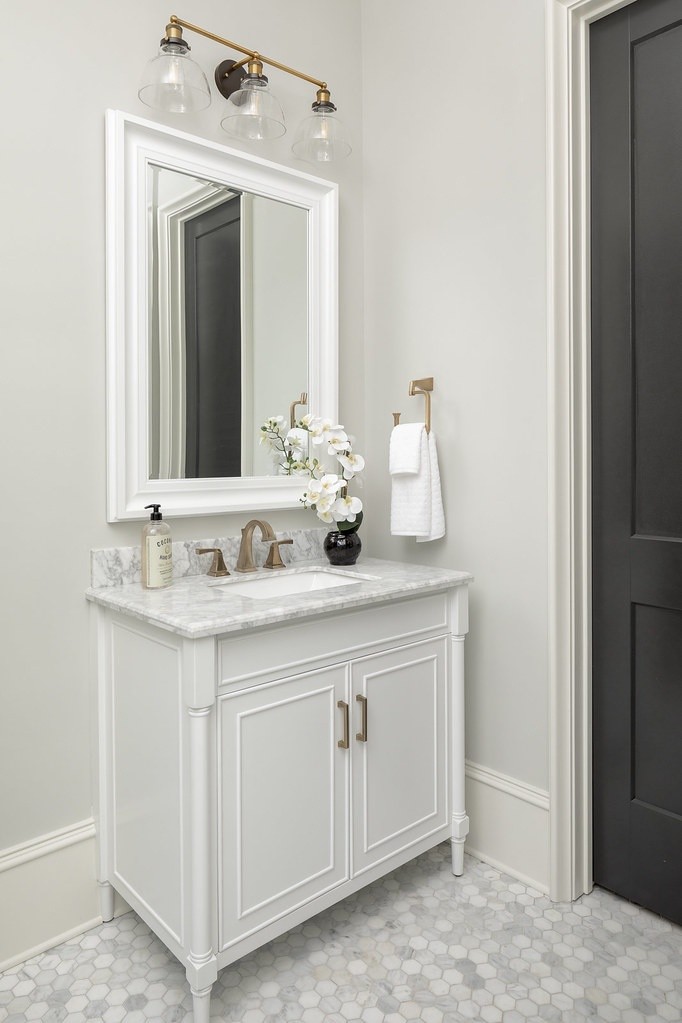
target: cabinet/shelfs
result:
[95,584,474,1023]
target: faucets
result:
[234,519,278,574]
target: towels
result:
[389,423,445,543]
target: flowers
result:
[258,417,307,479]
[301,414,364,532]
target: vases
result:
[324,531,362,566]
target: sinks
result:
[207,565,382,598]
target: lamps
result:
[137,12,354,164]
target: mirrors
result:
[100,106,337,527]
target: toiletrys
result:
[142,503,175,592]
